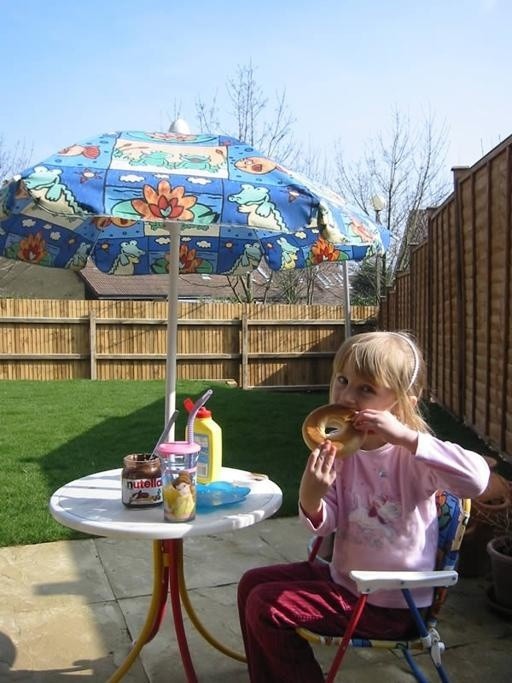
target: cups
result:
[154,440,203,522]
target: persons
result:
[163,471,195,522]
[237,331,490,682]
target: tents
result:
[0,117,400,450]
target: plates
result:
[194,481,251,511]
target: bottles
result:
[181,398,222,484]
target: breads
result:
[302,402,367,458]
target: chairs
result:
[296,472,473,683]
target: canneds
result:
[121,454,163,507]
